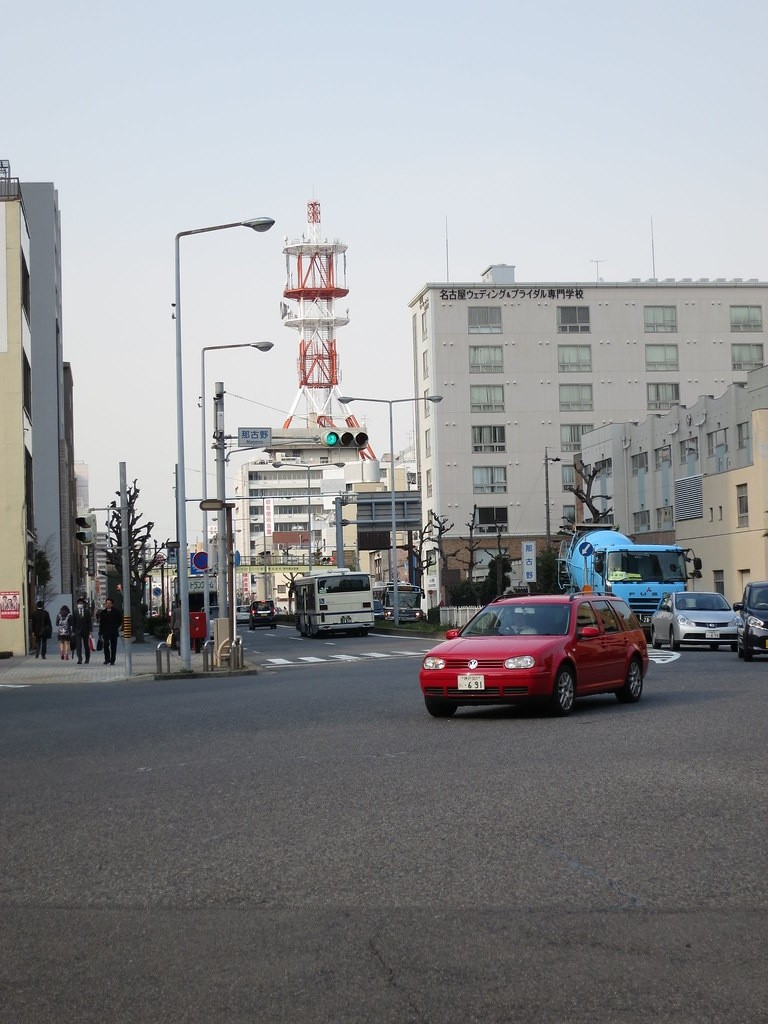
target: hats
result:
[77,597,86,603]
[661,591,670,600]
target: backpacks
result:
[56,613,71,636]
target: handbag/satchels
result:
[69,636,77,651]
[38,611,52,639]
[166,632,174,648]
[83,634,97,652]
[96,634,103,651]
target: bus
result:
[288,568,375,639]
[372,581,425,624]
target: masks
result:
[76,604,85,609]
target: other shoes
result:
[33,652,117,668]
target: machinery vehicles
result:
[556,523,702,644]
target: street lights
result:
[200,341,275,641]
[175,217,277,671]
[272,462,345,571]
[338,395,443,623]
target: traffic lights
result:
[75,513,97,545]
[321,431,369,447]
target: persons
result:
[0,596,19,610]
[500,606,567,635]
[169,601,198,655]
[31,601,51,660]
[56,605,73,660]
[99,598,122,665]
[72,598,93,665]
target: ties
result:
[515,628,524,635]
[80,610,82,616]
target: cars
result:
[201,606,228,640]
[419,591,649,717]
[650,591,740,651]
[373,600,385,620]
[236,605,251,621]
[249,600,277,630]
[732,580,767,662]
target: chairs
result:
[696,598,714,609]
[757,589,768,603]
